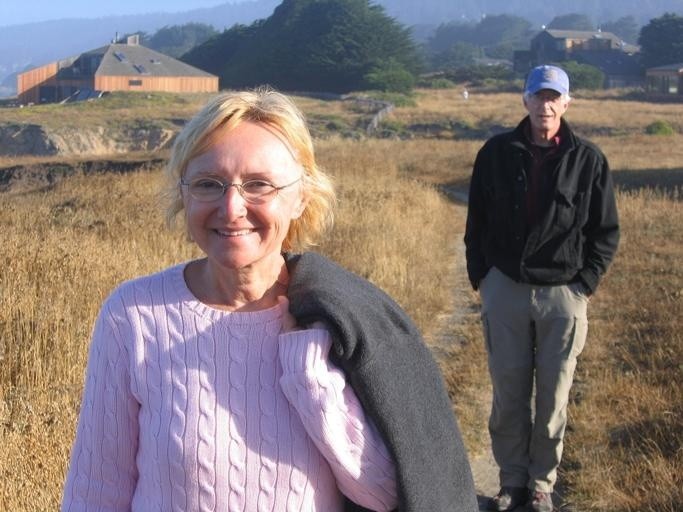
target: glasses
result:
[178,175,303,204]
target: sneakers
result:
[528,489,554,512]
[486,487,530,512]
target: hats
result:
[523,64,569,95]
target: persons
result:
[464,62,620,510]
[60,85,405,512]
